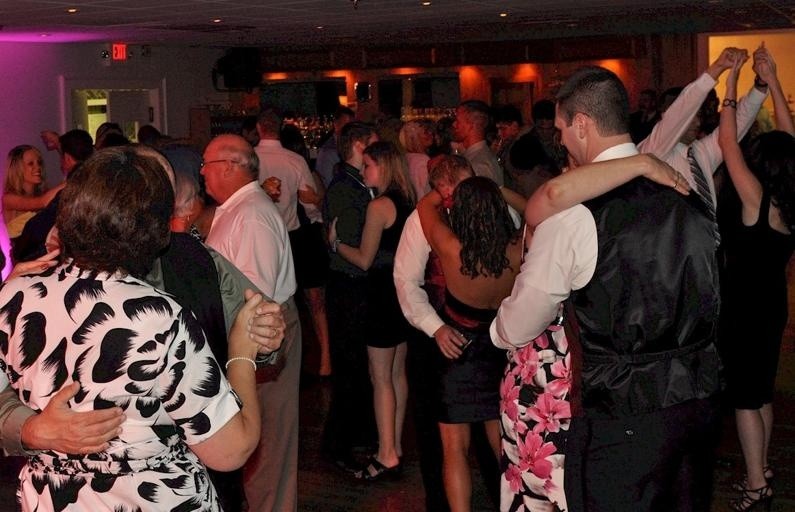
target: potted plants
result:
[128,120,134,138]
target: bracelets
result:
[720,98,738,108]
[223,356,257,370]
[331,239,340,252]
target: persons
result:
[492,110,531,153]
[201,134,302,511]
[504,100,569,197]
[501,134,560,197]
[92,122,135,146]
[636,48,776,286]
[393,156,525,511]
[3,145,67,239]
[9,130,92,268]
[255,109,324,291]
[140,125,202,187]
[316,107,355,189]
[490,141,691,512]
[170,165,206,240]
[632,90,661,143]
[323,120,393,482]
[401,120,436,200]
[417,176,523,510]
[452,102,510,188]
[717,50,795,511]
[491,66,727,511]
[329,142,416,481]
[434,117,463,154]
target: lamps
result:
[351,0,360,9]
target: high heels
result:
[731,463,775,494]
[728,484,777,512]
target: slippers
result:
[350,458,403,483]
[366,452,405,467]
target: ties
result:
[685,146,723,250]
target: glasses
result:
[200,158,240,167]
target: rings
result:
[272,327,280,340]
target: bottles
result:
[417,107,425,119]
[424,106,430,120]
[284,111,337,158]
[412,108,417,120]
[448,108,457,116]
[436,107,449,121]
[430,106,436,120]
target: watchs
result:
[754,75,770,89]
